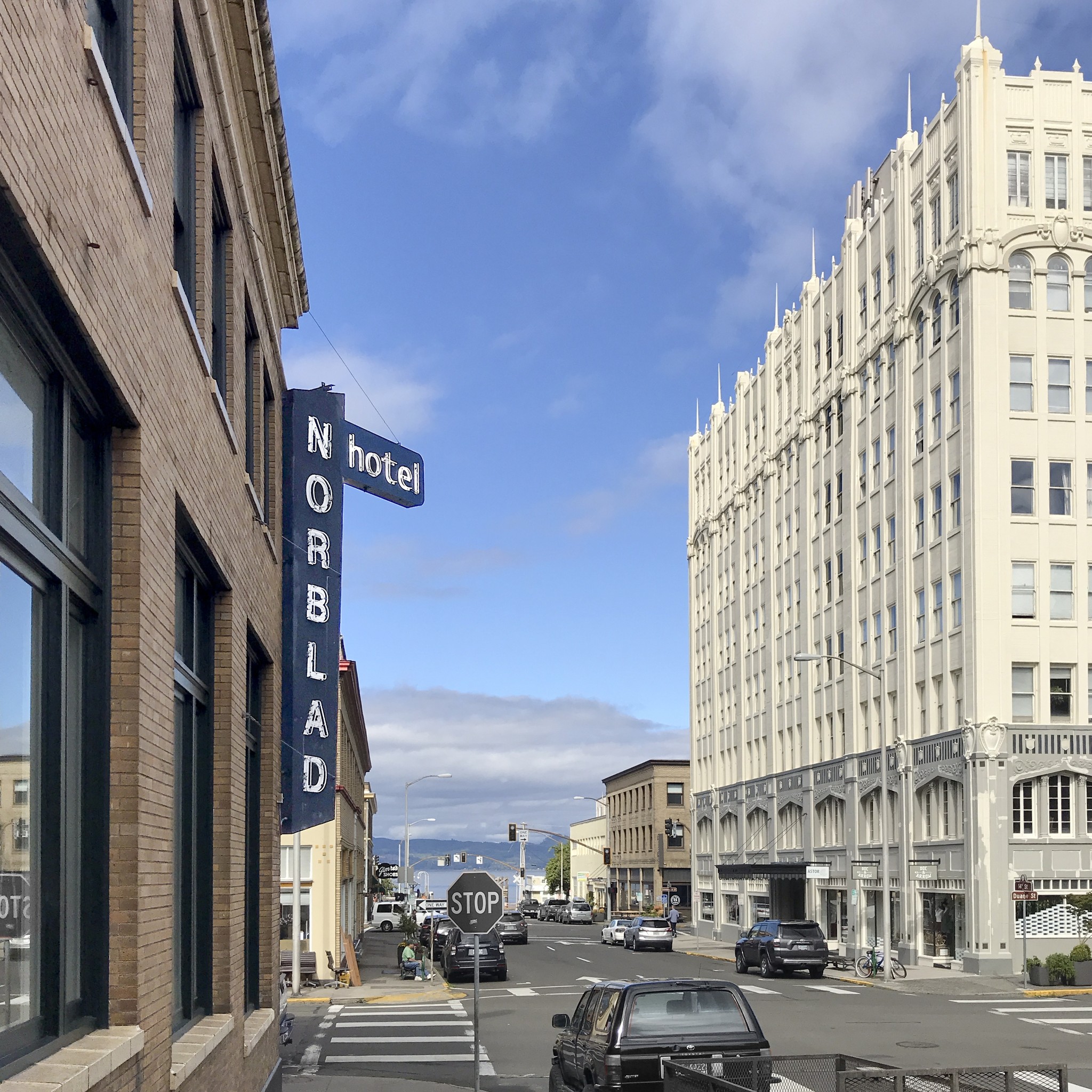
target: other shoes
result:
[417,936,420,939]
[672,936,674,938]
[414,976,422,981]
[427,974,436,980]
[674,933,677,938]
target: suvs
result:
[549,976,783,1092]
[734,919,830,978]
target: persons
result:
[409,909,420,938]
[401,940,436,981]
[373,893,378,902]
[664,905,679,937]
[934,903,944,933]
[940,901,951,933]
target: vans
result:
[371,900,444,933]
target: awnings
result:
[371,875,386,893]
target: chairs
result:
[666,999,688,1016]
[323,951,351,989]
[280,950,319,988]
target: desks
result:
[677,912,685,923]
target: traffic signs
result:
[415,899,448,912]
[1011,875,1039,902]
[661,886,677,892]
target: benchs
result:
[682,915,688,920]
[399,963,415,980]
[826,955,855,971]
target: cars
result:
[441,926,508,984]
[624,916,673,952]
[601,919,634,946]
[515,897,593,924]
[426,918,452,961]
[419,915,449,948]
[494,910,528,945]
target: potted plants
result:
[1060,890,1092,986]
[388,901,425,967]
[1021,952,1076,986]
[591,908,606,922]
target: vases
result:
[924,931,947,957]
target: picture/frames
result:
[938,947,950,957]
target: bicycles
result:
[867,951,907,978]
[855,941,896,979]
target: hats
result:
[408,939,417,944]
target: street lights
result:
[790,654,893,982]
[398,773,530,941]
[573,796,612,922]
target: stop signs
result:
[446,871,505,935]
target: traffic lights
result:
[460,852,466,863]
[520,868,524,877]
[508,823,516,841]
[665,817,672,837]
[603,848,611,865]
[608,887,618,894]
